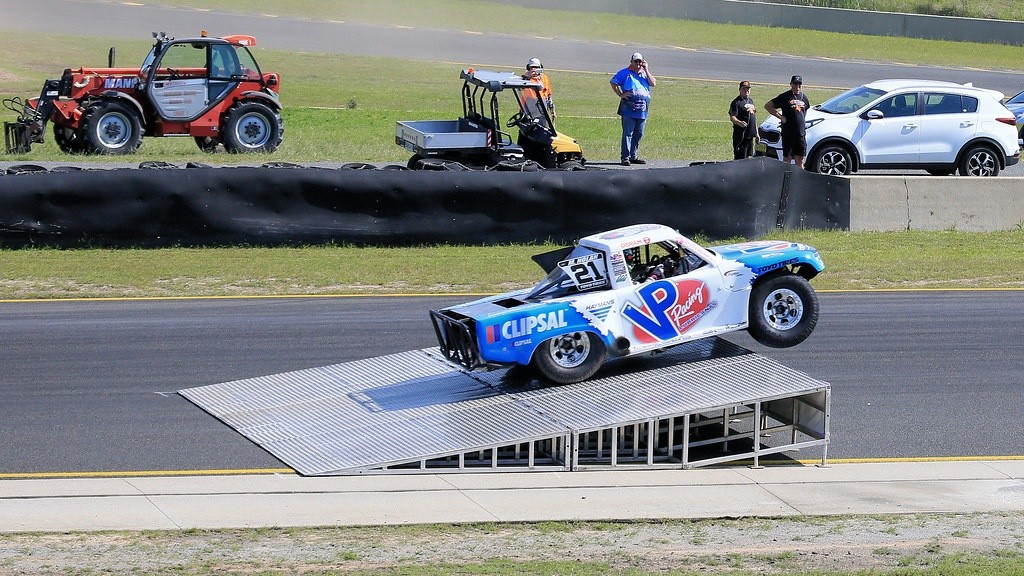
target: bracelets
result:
[619,93,622,96]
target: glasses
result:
[794,82,802,85]
[634,60,641,63]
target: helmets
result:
[623,250,634,272]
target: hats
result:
[631,53,643,61]
[529,58,541,67]
[739,81,752,89]
[791,75,802,83]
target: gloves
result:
[640,265,655,279]
[650,264,664,278]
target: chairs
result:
[886,96,909,116]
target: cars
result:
[1004,89,1024,149]
[430,223,824,387]
[754,77,1021,177]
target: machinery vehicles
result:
[1,30,284,157]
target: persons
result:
[610,53,657,165]
[626,251,664,284]
[728,81,761,159]
[764,75,810,167]
[518,57,556,144]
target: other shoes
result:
[621,159,631,166]
[630,158,646,164]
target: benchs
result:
[470,110,526,158]
